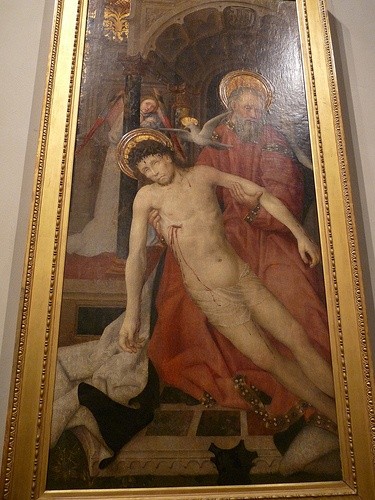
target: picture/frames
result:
[2,0,374,500]
